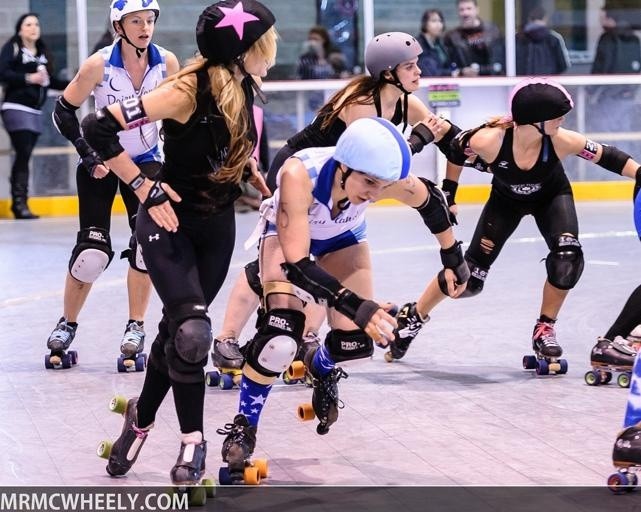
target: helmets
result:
[112,0,160,34]
[197,0,276,62]
[367,32,424,78]
[335,117,412,180]
[509,79,574,125]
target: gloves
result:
[441,241,470,285]
[337,293,383,330]
[442,178,459,210]
[473,154,493,172]
[141,181,171,210]
[80,142,103,177]
[406,123,433,155]
[242,159,260,184]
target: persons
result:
[296,28,352,79]
[377,84,641,378]
[586,166,641,387]
[607,348,640,493]
[45,0,179,373]
[0,14,52,220]
[589,5,641,75]
[414,8,478,76]
[516,5,571,74]
[81,0,279,508]
[444,0,503,75]
[218,117,469,488]
[206,32,464,392]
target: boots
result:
[9,172,39,219]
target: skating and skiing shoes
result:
[287,347,348,435]
[283,327,321,388]
[523,315,568,375]
[96,395,155,479]
[117,319,148,372]
[44,318,79,368]
[607,421,641,495]
[169,431,216,507]
[627,332,641,350]
[376,301,431,363]
[585,336,638,387]
[217,414,269,484]
[206,330,245,390]
